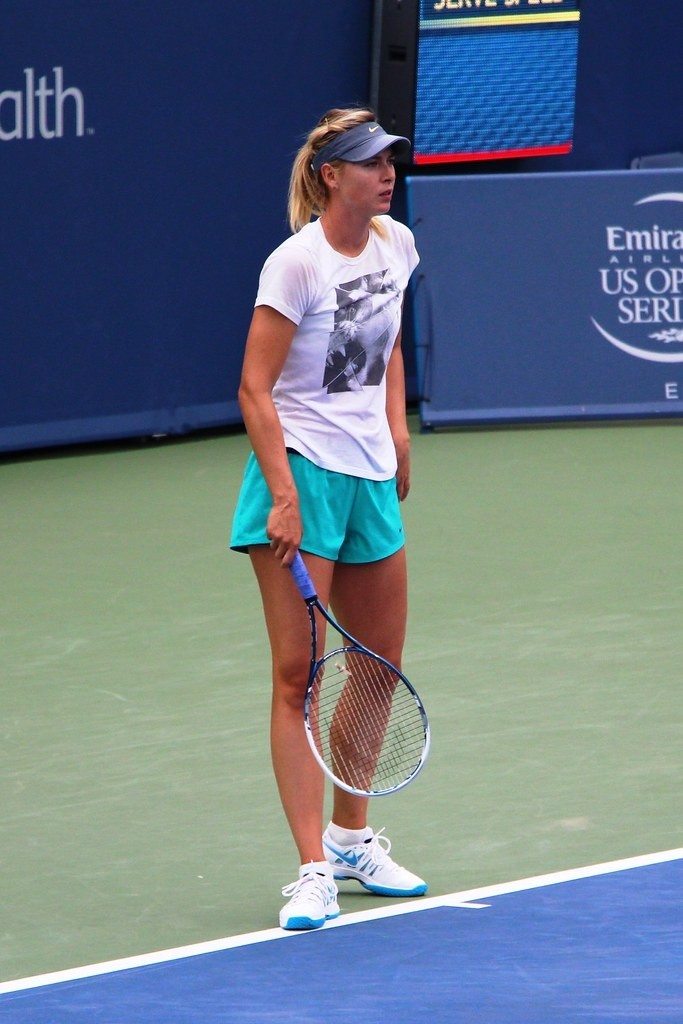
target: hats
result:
[311,122,411,173]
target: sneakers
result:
[280,860,340,929]
[320,827,428,897]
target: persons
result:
[228,104,429,929]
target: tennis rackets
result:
[290,550,432,798]
